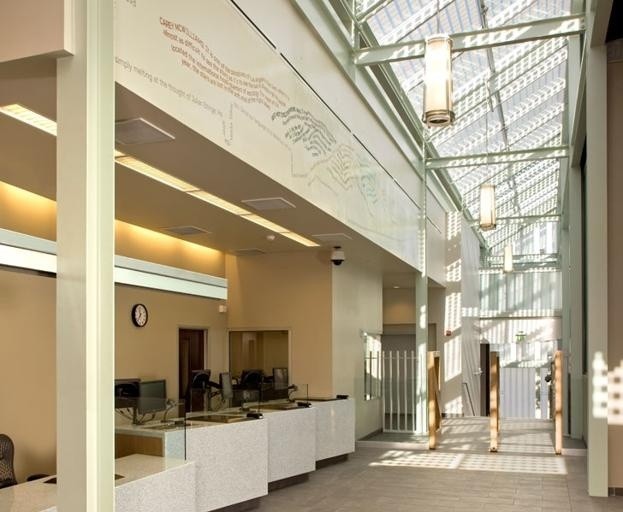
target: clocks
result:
[132,304,148,327]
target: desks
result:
[0,393,356,512]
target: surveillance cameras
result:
[330,250,345,265]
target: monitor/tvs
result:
[186,369,212,396]
[271,367,289,391]
[240,368,263,390]
[220,372,235,400]
[115,378,141,409]
[135,379,167,415]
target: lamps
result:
[480,49,497,231]
[421,0,453,127]
[504,173,512,272]
